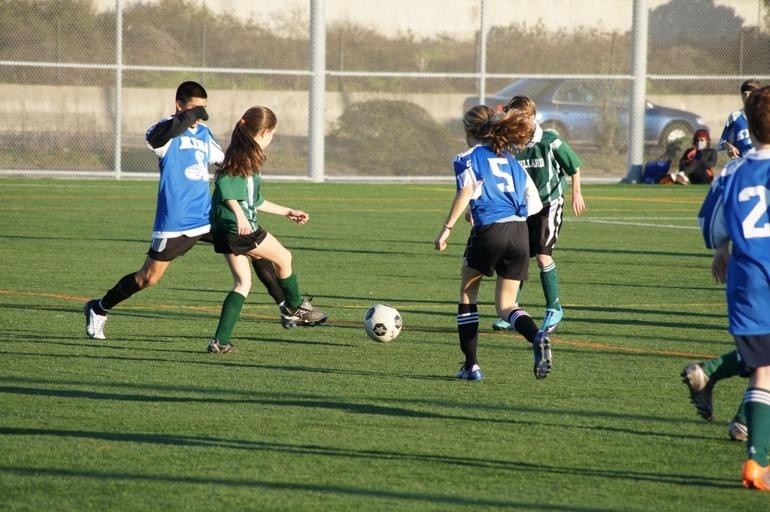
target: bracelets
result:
[443,222,454,232]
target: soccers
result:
[363,303,403,343]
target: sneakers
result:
[83,299,109,340]
[279,305,327,328]
[683,362,715,423]
[544,306,564,341]
[744,458,770,491]
[493,319,517,335]
[208,337,234,359]
[533,331,553,386]
[454,364,482,384]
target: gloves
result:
[182,106,209,125]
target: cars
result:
[462,79,710,153]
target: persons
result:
[434,105,553,381]
[681,348,751,442]
[207,106,328,353]
[717,79,762,158]
[490,94,585,333]
[675,128,717,186]
[698,84,769,491]
[82,81,317,339]
